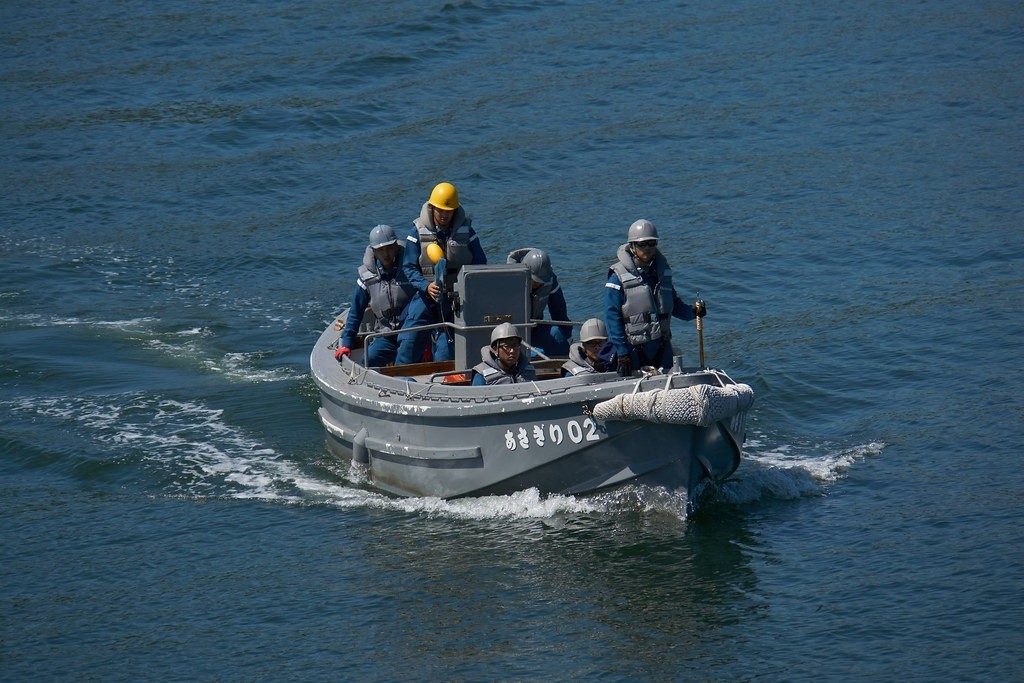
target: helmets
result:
[626,219,660,242]
[521,249,551,283]
[579,318,608,343]
[490,322,523,350]
[369,224,396,249]
[428,182,460,211]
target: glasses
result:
[636,241,657,247]
[500,343,520,350]
[586,341,605,347]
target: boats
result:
[307,263,756,514]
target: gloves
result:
[335,346,351,362]
[617,356,632,376]
[694,300,706,318]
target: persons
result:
[392,184,489,366]
[333,225,453,367]
[607,219,707,377]
[470,248,617,386]
